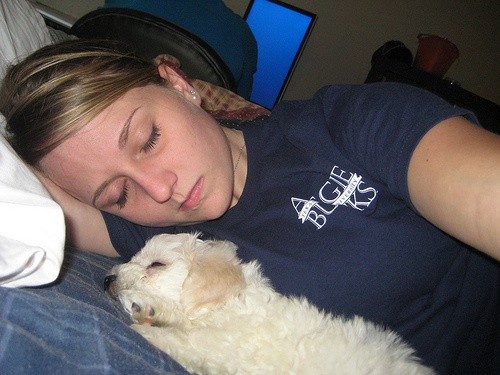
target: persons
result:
[0,36,500,375]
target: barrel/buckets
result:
[413,33,459,78]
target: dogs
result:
[105,232,437,375]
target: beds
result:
[0,0,272,375]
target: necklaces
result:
[233,145,245,174]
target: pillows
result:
[0,1,84,289]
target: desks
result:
[362,38,499,136]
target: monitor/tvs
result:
[242,0,319,111]
